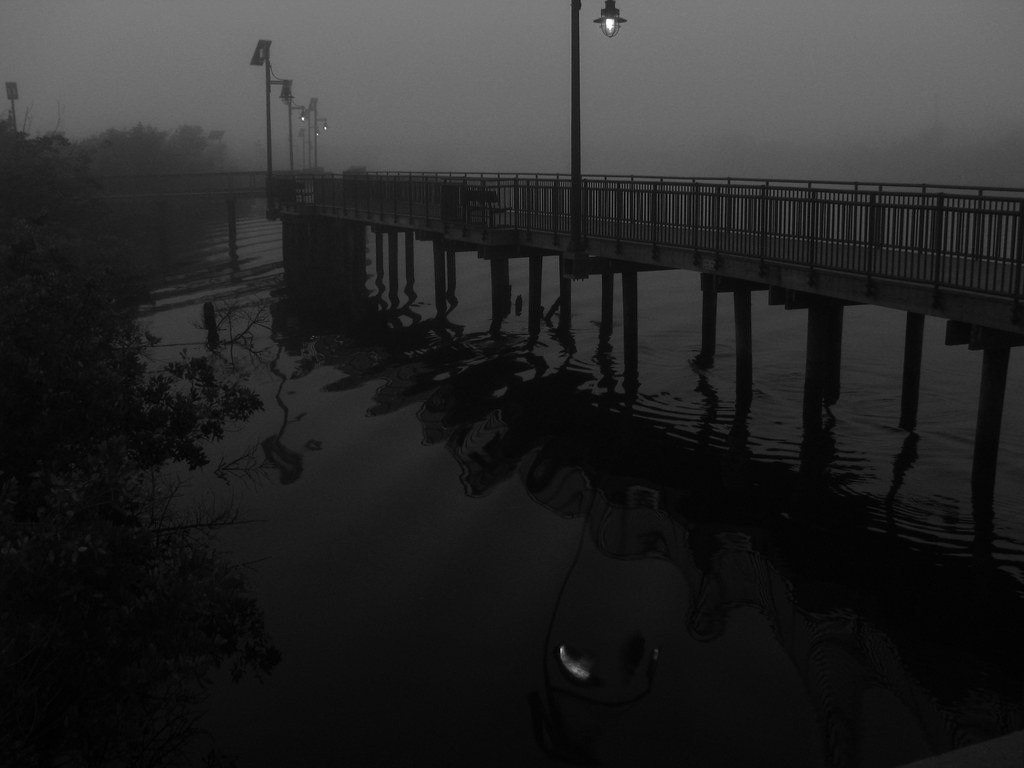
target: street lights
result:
[563,0,628,254]
[305,97,329,171]
[249,39,297,207]
[284,80,310,173]
